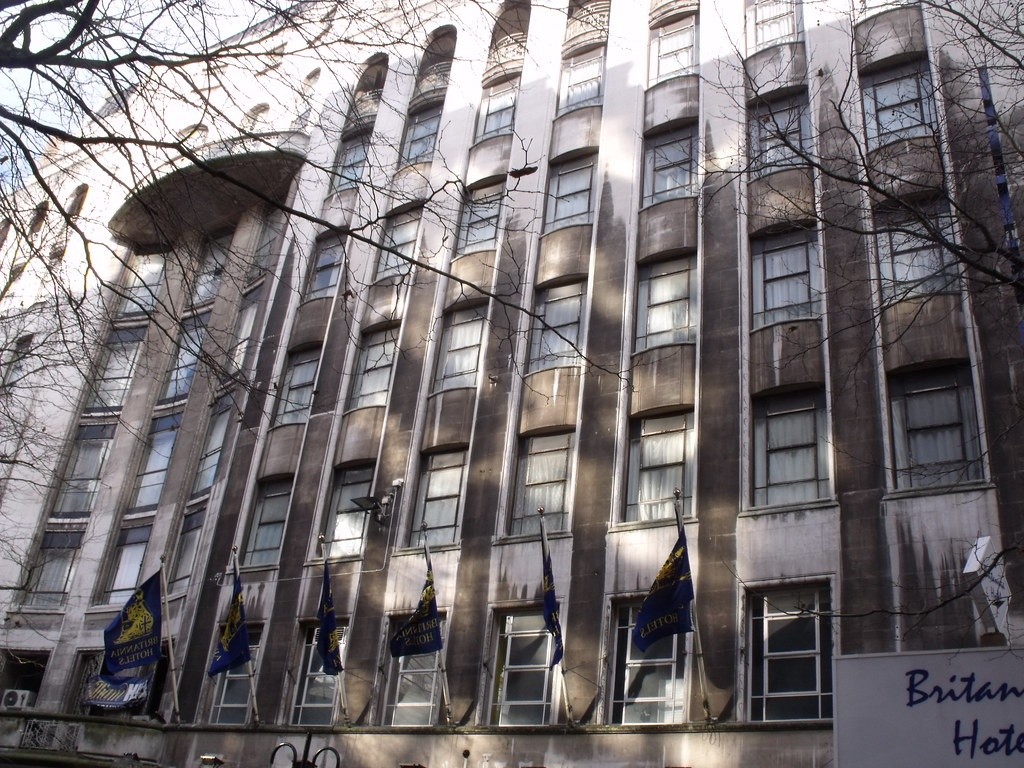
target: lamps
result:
[349,496,381,514]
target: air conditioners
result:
[1,689,37,710]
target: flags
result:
[542,550,563,671]
[207,564,251,677]
[103,571,162,674]
[389,562,443,658]
[632,528,695,653]
[316,560,343,677]
